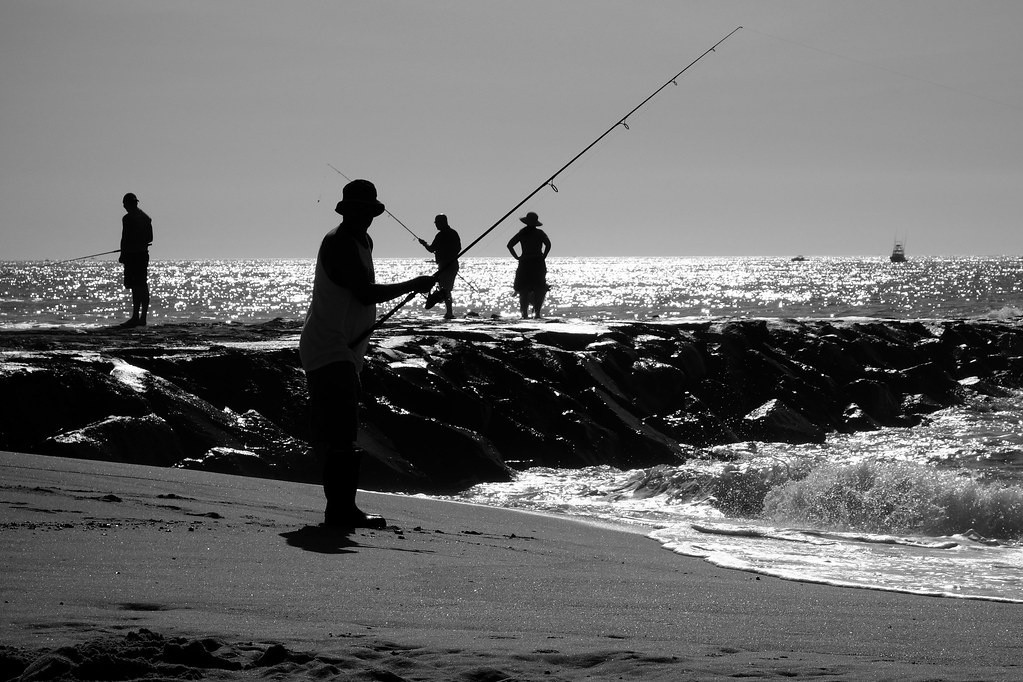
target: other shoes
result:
[353,510,387,530]
[120,314,139,326]
[523,316,533,319]
[534,316,544,319]
[323,512,356,535]
[443,313,455,319]
[139,316,147,326]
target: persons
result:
[117,193,153,329]
[418,214,461,321]
[301,178,436,529]
[506,211,552,320]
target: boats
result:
[791,254,808,262]
[889,228,910,263]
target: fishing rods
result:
[349,27,742,350]
[326,161,476,289]
[54,242,150,264]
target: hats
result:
[519,212,543,227]
[335,180,385,218]
[123,193,139,203]
